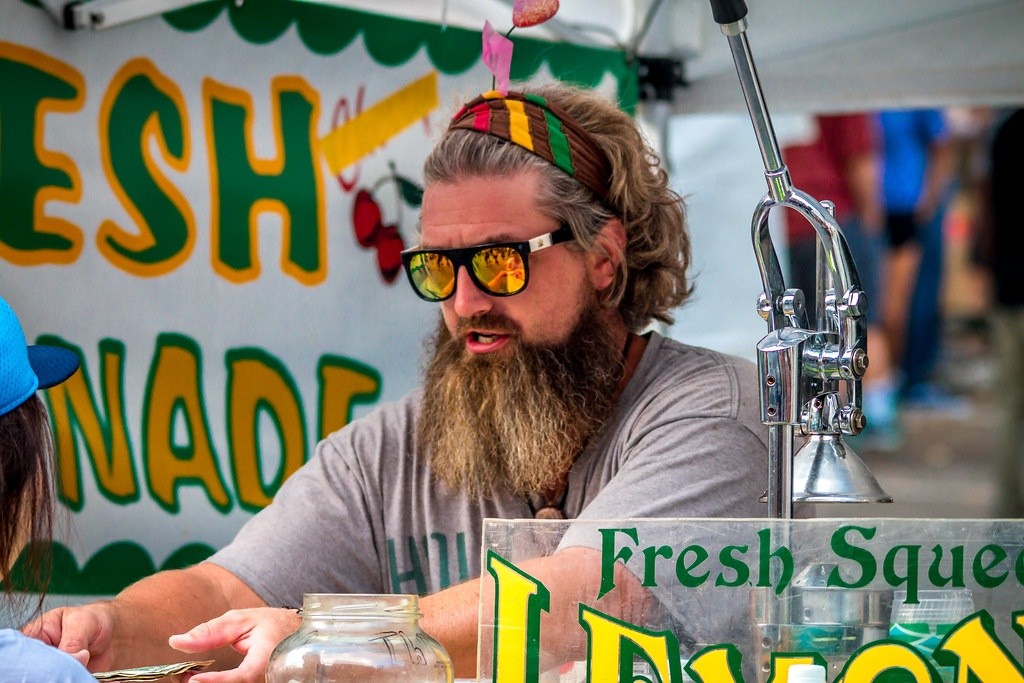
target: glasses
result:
[402,225,582,302]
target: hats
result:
[0,295,80,414]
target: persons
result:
[965,106,1024,522]
[0,294,100,682]
[786,105,972,454]
[20,84,817,683]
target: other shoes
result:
[843,414,905,455]
[901,379,968,417]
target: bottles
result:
[264,593,454,683]
